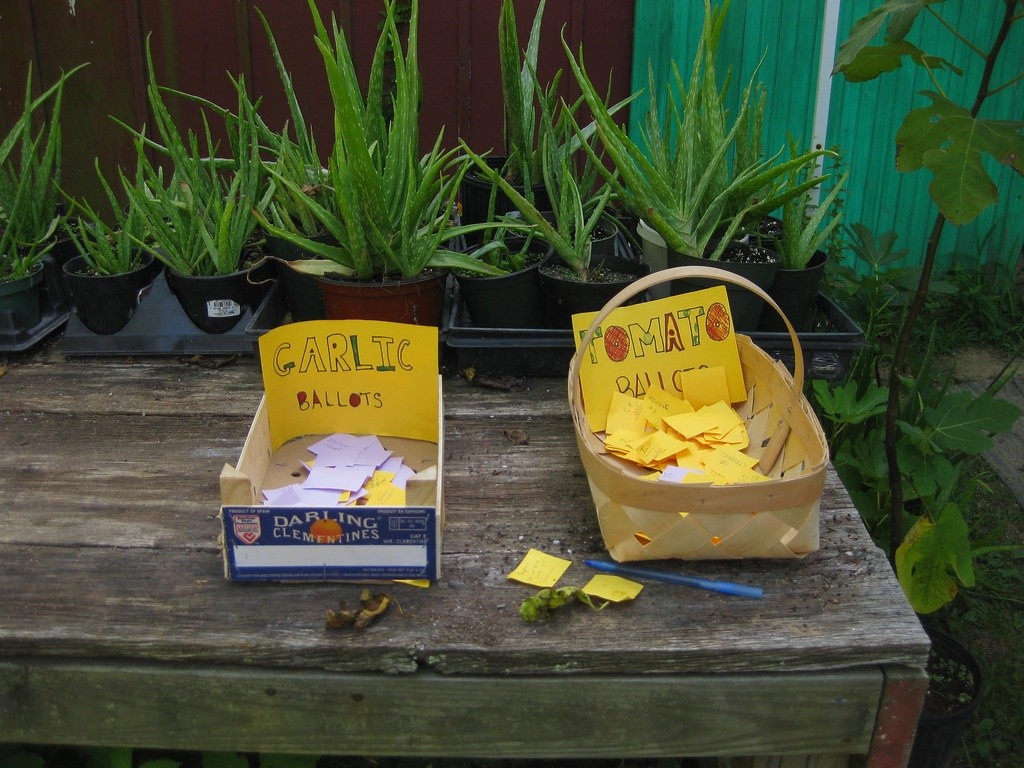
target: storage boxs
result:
[217,374,444,583]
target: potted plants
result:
[440,165,555,329]
[507,68,644,255]
[248,0,540,329]
[0,121,60,330]
[663,59,808,249]
[445,65,648,331]
[809,376,1024,768]
[461,0,644,240]
[616,121,642,254]
[756,130,851,334]
[0,59,92,302]
[278,123,346,321]
[559,0,839,329]
[123,29,268,333]
[52,121,155,335]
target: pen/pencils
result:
[581,559,763,600]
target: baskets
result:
[569,266,830,562]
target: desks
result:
[0,327,934,768]
